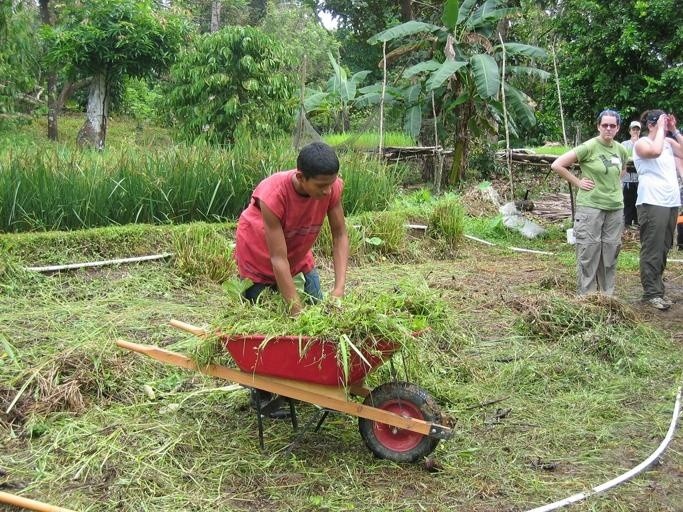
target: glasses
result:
[602,123,616,128]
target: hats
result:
[629,120,641,129]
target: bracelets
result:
[671,129,678,138]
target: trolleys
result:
[116,314,459,461]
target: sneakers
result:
[644,296,676,309]
[250,390,290,418]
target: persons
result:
[232,142,353,417]
[631,106,682,312]
[673,154,682,251]
[549,108,628,297]
[619,120,641,228]
